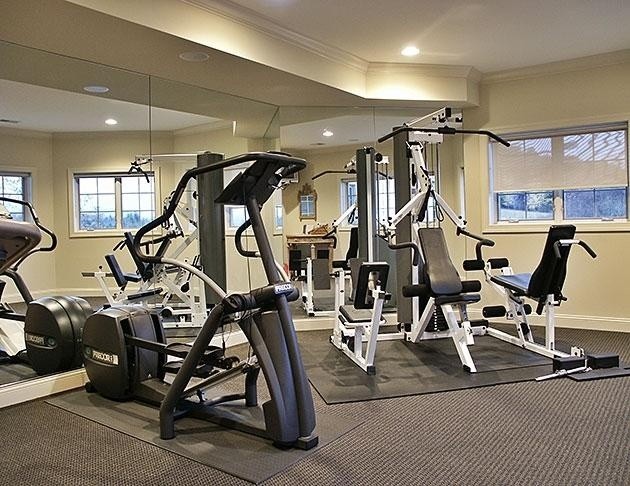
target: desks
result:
[283,234,335,282]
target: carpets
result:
[296,327,589,406]
[80,296,224,337]
[42,374,366,485]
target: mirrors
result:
[297,181,318,222]
